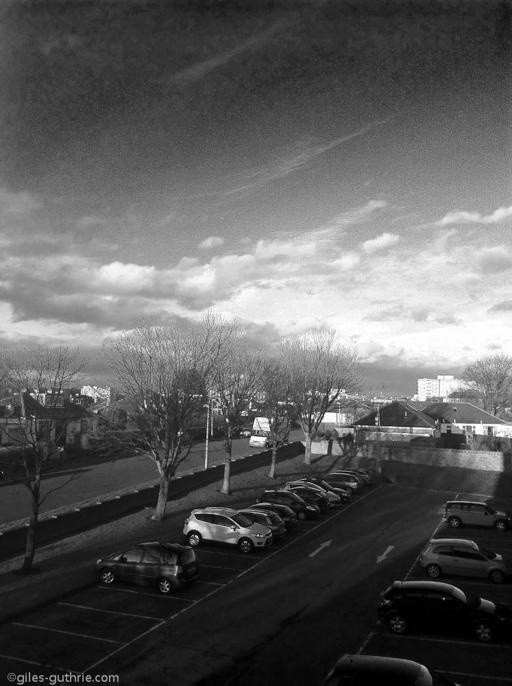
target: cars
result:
[240,427,252,439]
[94,539,199,594]
[322,652,432,686]
[238,462,375,544]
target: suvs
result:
[375,581,505,641]
[418,539,508,583]
[181,504,273,553]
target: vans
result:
[444,500,510,531]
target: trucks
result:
[249,415,289,446]
